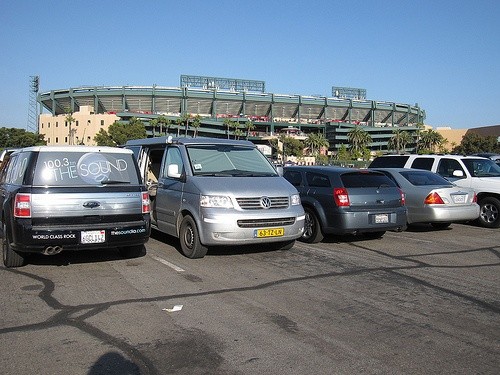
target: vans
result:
[127,138,306,258]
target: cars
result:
[362,168,479,232]
[282,167,407,244]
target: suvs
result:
[2,143,151,265]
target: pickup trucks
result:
[370,154,500,228]
